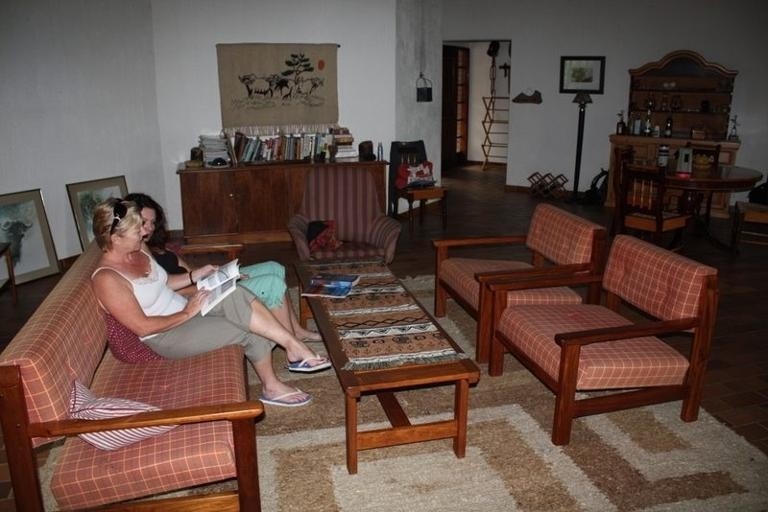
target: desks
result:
[175,155,389,247]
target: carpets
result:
[34,269,768,512]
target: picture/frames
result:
[560,56,604,94]
[65,175,129,251]
[0,188,61,288]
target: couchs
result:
[0,241,264,511]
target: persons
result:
[90,195,333,408]
[124,191,323,341]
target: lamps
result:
[565,93,593,203]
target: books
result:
[196,122,360,168]
[299,271,362,300]
[196,257,241,317]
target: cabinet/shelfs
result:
[604,49,740,219]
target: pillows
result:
[396,160,434,188]
[68,379,179,450]
[105,314,161,362]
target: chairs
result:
[429,202,606,363]
[287,166,402,265]
[734,200,768,249]
[616,162,692,249]
[609,145,672,237]
[390,140,447,232]
[486,233,720,446]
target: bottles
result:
[658,144,670,167]
[377,142,383,161]
[646,91,680,112]
[615,110,672,138]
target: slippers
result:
[258,387,312,407]
[288,355,331,372]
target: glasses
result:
[109,198,126,234]
